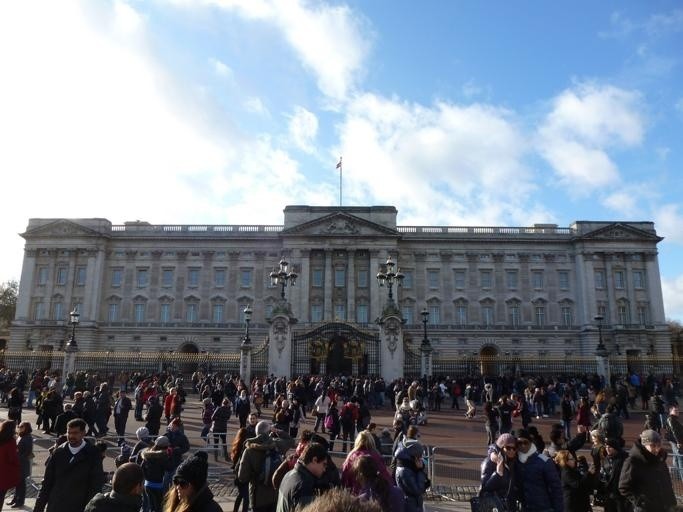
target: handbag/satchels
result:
[312,405,319,416]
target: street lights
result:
[594,312,609,383]
[267,255,298,378]
[420,308,433,377]
[377,256,406,382]
[64,306,80,379]
[239,304,252,384]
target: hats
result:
[121,443,133,456]
[135,427,149,437]
[641,429,663,446]
[169,417,182,427]
[173,451,208,490]
[402,440,424,457]
[514,428,529,439]
[255,421,269,436]
[605,434,625,450]
[495,434,516,448]
[155,435,169,449]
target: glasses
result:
[518,440,530,445]
[173,479,191,488]
[504,446,517,451]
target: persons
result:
[134,381,145,422]
[141,435,171,485]
[164,388,183,423]
[211,397,231,462]
[161,416,190,454]
[590,431,606,507]
[393,424,420,473]
[113,389,132,444]
[129,426,155,464]
[34,386,49,430]
[235,420,296,512]
[620,429,678,512]
[271,429,318,492]
[394,441,431,512]
[487,428,558,512]
[666,404,683,482]
[0,419,18,512]
[516,425,545,453]
[0,364,250,403]
[365,422,381,452]
[549,428,570,462]
[378,427,393,454]
[144,395,164,438]
[340,429,394,509]
[554,449,592,512]
[6,386,25,428]
[251,376,272,417]
[161,451,222,512]
[5,421,34,509]
[234,389,250,426]
[274,374,463,438]
[548,422,568,457]
[71,391,83,419]
[53,402,77,436]
[474,432,517,512]
[83,462,144,512]
[309,435,339,489]
[38,391,57,434]
[82,390,98,437]
[611,370,683,430]
[237,412,258,439]
[95,383,110,436]
[598,434,630,512]
[32,418,105,511]
[464,375,625,439]
[115,442,130,469]
[274,441,328,512]
[199,397,214,444]
[230,427,255,512]
[356,453,388,512]
